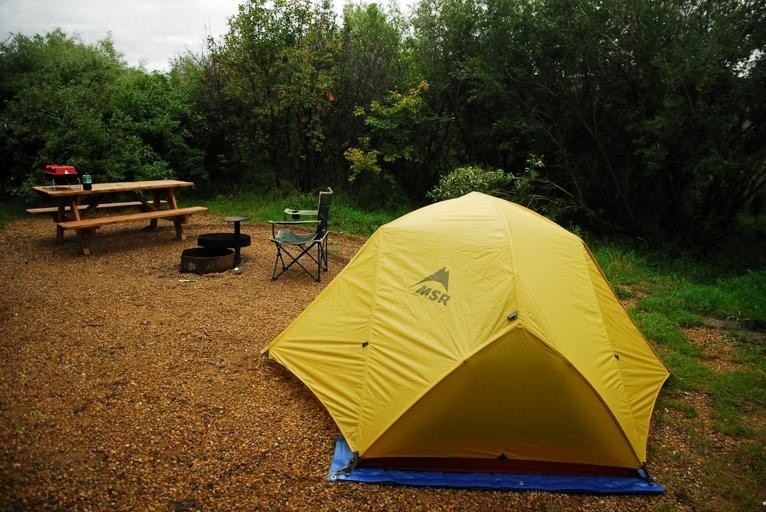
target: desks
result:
[32,177,194,256]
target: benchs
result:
[25,198,208,230]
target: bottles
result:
[82,171,92,190]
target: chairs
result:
[266,185,333,284]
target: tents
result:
[260,191,673,486]
